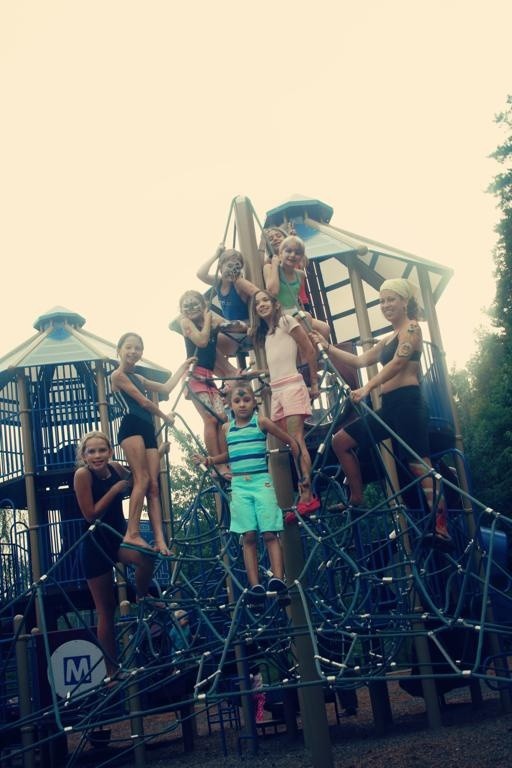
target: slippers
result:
[119,543,177,560]
[327,502,348,514]
[268,578,292,606]
[250,577,266,612]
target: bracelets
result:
[207,456,212,465]
[323,341,331,351]
[205,457,209,465]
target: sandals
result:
[424,531,453,542]
[210,463,232,479]
[284,496,320,526]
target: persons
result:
[308,275,454,547]
[72,429,172,691]
[260,222,289,266]
[190,380,302,606]
[195,239,278,395]
[176,288,252,486]
[260,233,337,385]
[251,288,323,526]
[108,329,199,559]
